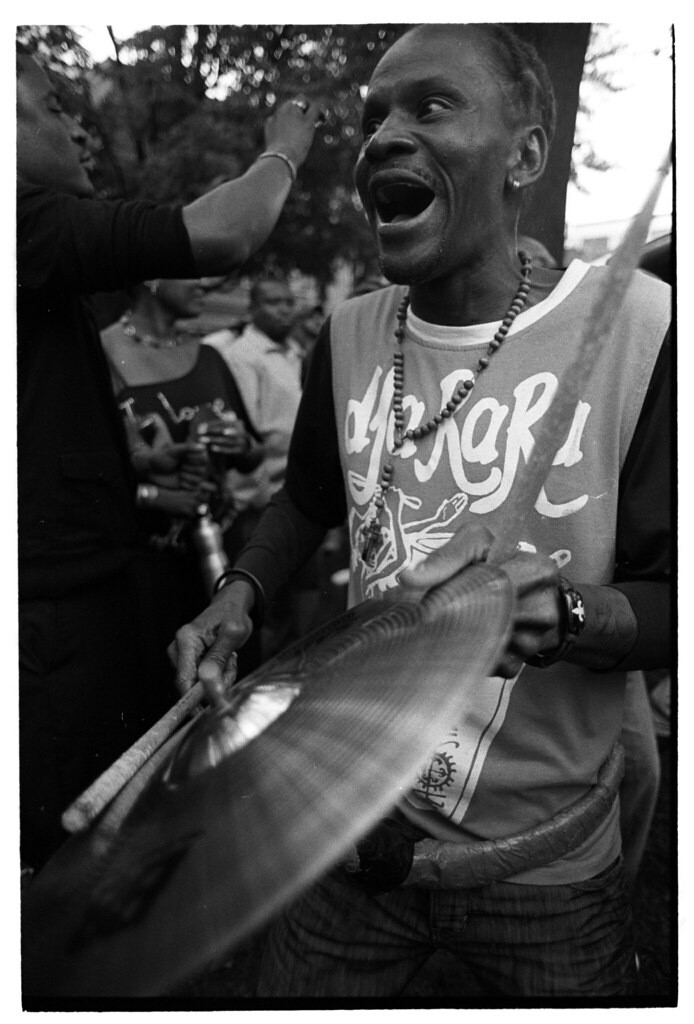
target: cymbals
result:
[18,563,514,1012]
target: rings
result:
[223,426,238,437]
[291,99,307,111]
[197,503,211,514]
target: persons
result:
[162,24,678,1008]
[18,41,328,897]
[98,273,266,636]
[180,276,327,376]
[226,273,303,496]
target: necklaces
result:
[361,245,535,558]
[121,307,199,350]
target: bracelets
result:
[212,567,266,627]
[258,150,297,183]
[140,482,154,508]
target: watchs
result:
[556,570,586,662]
